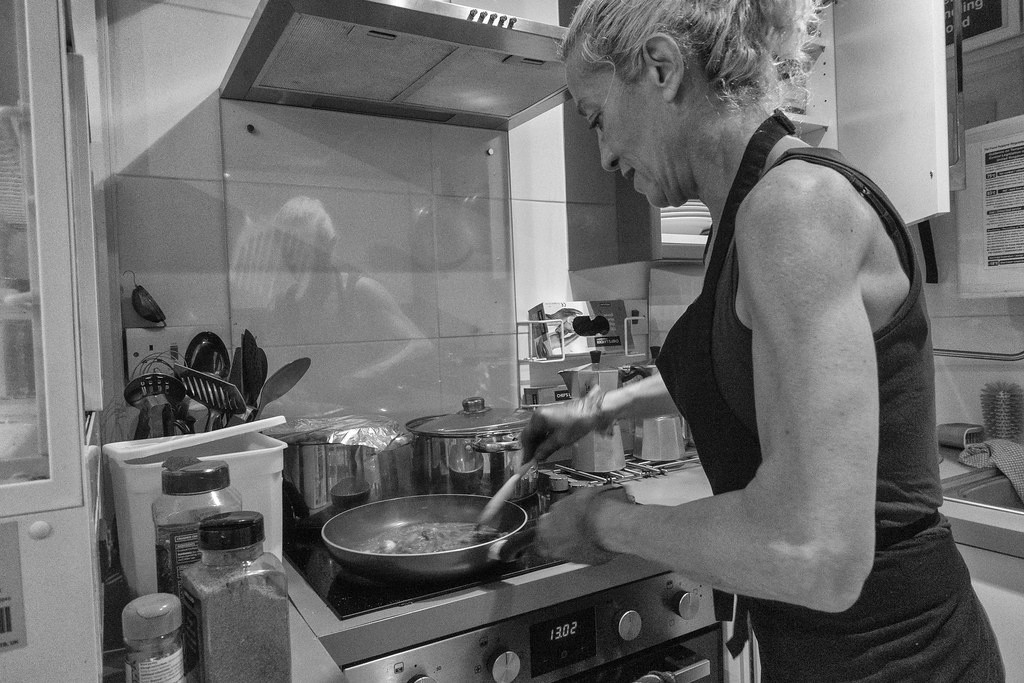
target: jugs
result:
[557,350,626,472]
[623,346,689,461]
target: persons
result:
[518,0,1007,682]
[231,195,435,401]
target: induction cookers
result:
[282,471,721,683]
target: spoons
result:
[254,357,311,420]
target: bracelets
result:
[578,483,634,557]
[584,383,615,438]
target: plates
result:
[661,199,712,235]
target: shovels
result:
[165,360,248,434]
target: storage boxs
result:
[101,414,288,598]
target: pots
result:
[405,397,541,502]
[322,482,538,580]
[282,435,413,527]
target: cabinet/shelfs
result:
[616,0,950,261]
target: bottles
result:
[152,460,243,594]
[123,594,188,683]
[181,511,291,683]
